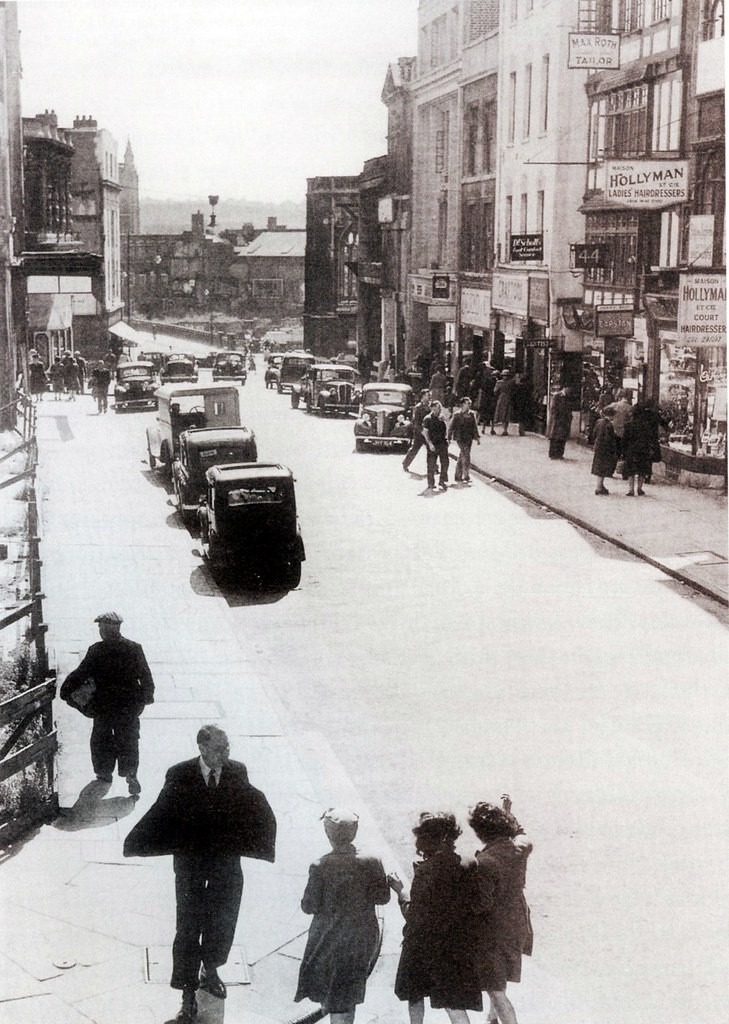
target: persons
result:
[547,383,574,460]
[386,811,483,1024]
[448,396,481,482]
[59,611,155,794]
[29,354,50,402]
[392,346,533,436]
[90,360,112,413]
[50,350,86,401]
[401,389,442,475]
[467,793,533,1024]
[293,808,390,1024]
[104,348,130,380]
[122,724,277,1024]
[591,388,671,496]
[422,401,450,490]
[358,344,373,388]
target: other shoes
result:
[464,475,470,481]
[126,773,142,794]
[455,476,464,481]
[436,469,441,474]
[625,492,634,496]
[502,432,508,436]
[638,489,645,495]
[96,774,112,782]
[402,462,409,472]
[439,481,448,488]
[482,430,485,434]
[595,487,609,495]
[491,430,496,435]
[428,485,436,488]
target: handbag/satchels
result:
[616,459,626,474]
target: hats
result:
[603,407,616,415]
[93,611,123,625]
[500,369,511,376]
[319,807,358,840]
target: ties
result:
[208,769,217,787]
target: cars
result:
[354,382,420,454]
[146,381,242,479]
[263,348,334,409]
[140,350,217,377]
[217,314,304,354]
[169,426,259,528]
[212,351,248,387]
[113,360,161,414]
[302,363,360,420]
[159,357,198,387]
[195,461,306,591]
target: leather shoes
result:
[176,1001,198,1024]
[200,965,227,999]
